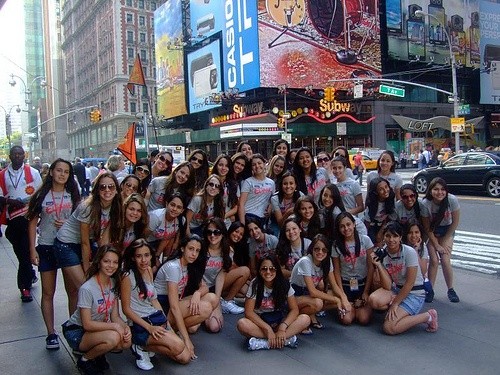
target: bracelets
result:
[282,322,288,328]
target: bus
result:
[137,145,185,169]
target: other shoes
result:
[21,276,38,303]
[301,310,327,334]
[425,309,438,333]
[425,291,434,302]
[448,288,460,303]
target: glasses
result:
[402,193,417,200]
[130,238,150,249]
[125,183,138,192]
[317,158,329,162]
[335,153,344,158]
[191,156,201,164]
[204,229,222,237]
[260,266,276,273]
[99,184,116,192]
[207,182,220,188]
[135,166,150,176]
[158,154,172,168]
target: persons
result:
[420,177,460,303]
[0,139,500,375]
[236,254,311,351]
[25,158,80,351]
[153,234,219,359]
[367,224,439,335]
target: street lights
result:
[9,73,48,165]
[415,10,460,153]
[0,105,21,157]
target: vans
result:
[81,158,107,167]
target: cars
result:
[411,152,500,198]
[348,151,378,171]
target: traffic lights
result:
[277,117,281,129]
[324,87,330,101]
[90,111,95,122]
[96,111,101,122]
[330,87,336,102]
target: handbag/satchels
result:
[353,166,358,175]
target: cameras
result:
[373,247,388,262]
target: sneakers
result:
[247,336,271,350]
[130,344,155,370]
[46,334,59,349]
[220,297,245,314]
[285,334,300,349]
[76,353,110,375]
